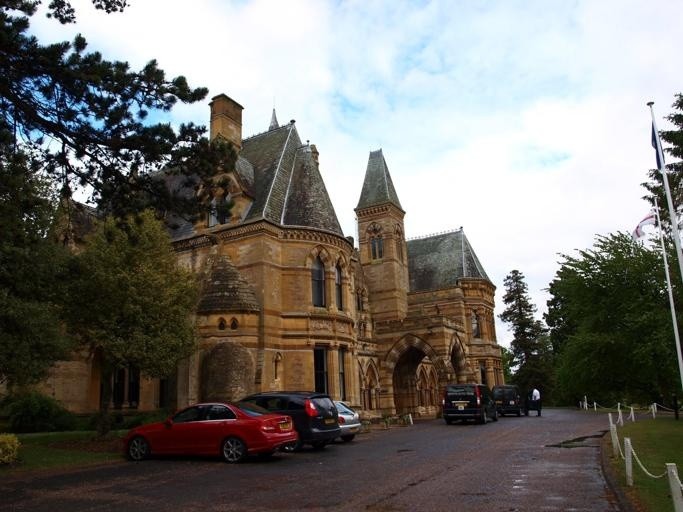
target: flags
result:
[632,214,656,239]
[650,121,665,172]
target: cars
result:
[332,399,360,442]
[113,398,297,465]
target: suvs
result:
[491,384,541,416]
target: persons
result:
[531,385,541,416]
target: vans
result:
[442,382,499,423]
[236,391,342,452]
